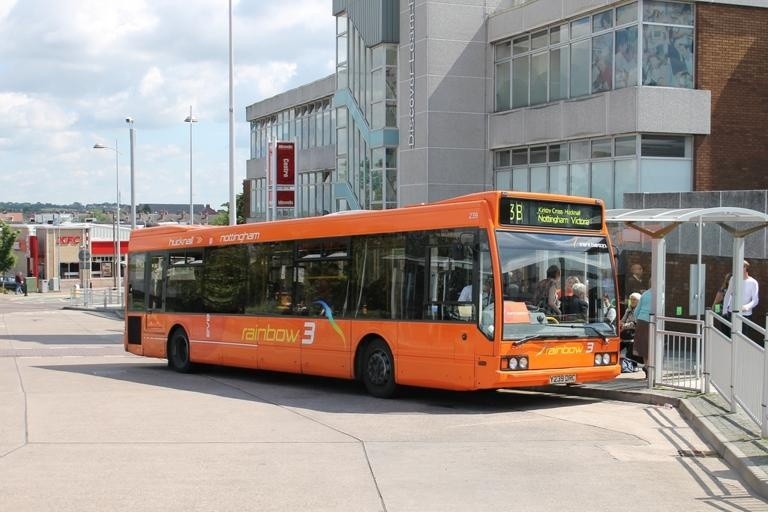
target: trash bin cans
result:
[38,280,48,293]
[25,277,37,293]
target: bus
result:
[122,190,623,399]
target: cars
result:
[0,274,24,292]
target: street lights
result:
[92,143,126,306]
[184,105,202,227]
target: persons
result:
[564,282,588,322]
[620,292,643,373]
[326,262,343,290]
[722,260,759,339]
[627,262,645,294]
[712,272,732,337]
[602,293,617,336]
[457,271,492,322]
[532,264,562,324]
[14,270,24,294]
[556,275,580,299]
[591,5,693,93]
[634,277,652,378]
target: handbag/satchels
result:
[621,355,637,373]
[535,280,562,322]
[620,328,637,340]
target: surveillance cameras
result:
[125,116,132,123]
